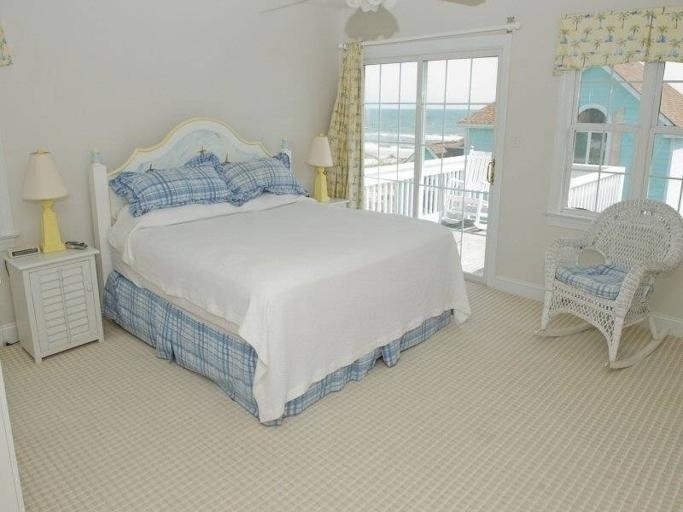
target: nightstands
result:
[331,197,351,208]
[3,243,104,365]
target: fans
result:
[256,0,488,44]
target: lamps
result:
[304,132,334,202]
[21,146,69,254]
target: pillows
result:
[108,151,234,217]
[220,146,311,206]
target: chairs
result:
[534,199,683,369]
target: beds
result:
[86,116,473,428]
